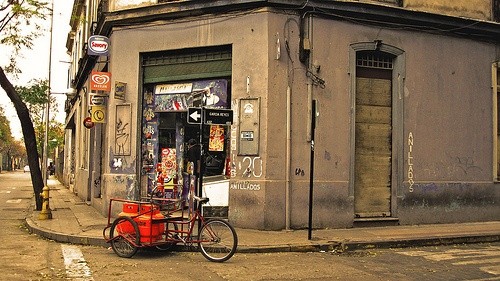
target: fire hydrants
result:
[38,184,52,219]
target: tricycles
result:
[102,183,238,263]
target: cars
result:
[23,165,30,172]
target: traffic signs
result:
[204,108,233,126]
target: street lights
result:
[43,87,77,187]
